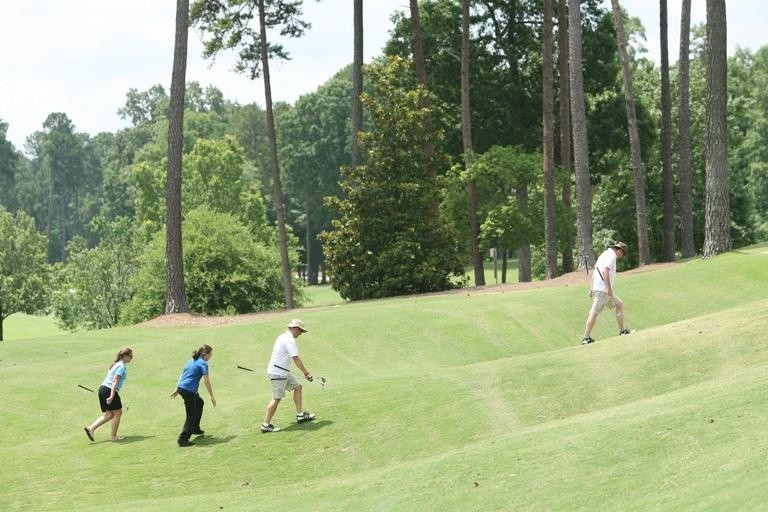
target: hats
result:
[287,319,308,332]
[615,242,629,255]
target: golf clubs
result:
[78,385,129,411]
[597,267,621,317]
[269,365,326,383]
[237,365,268,377]
[274,365,325,391]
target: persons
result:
[260,319,316,432]
[83,347,133,442]
[581,241,636,344]
[170,345,216,447]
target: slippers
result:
[84,427,94,441]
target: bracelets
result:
[304,372,309,377]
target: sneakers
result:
[582,336,595,344]
[620,328,636,336]
[260,423,280,432]
[297,411,315,424]
[192,429,204,434]
[178,438,194,445]
